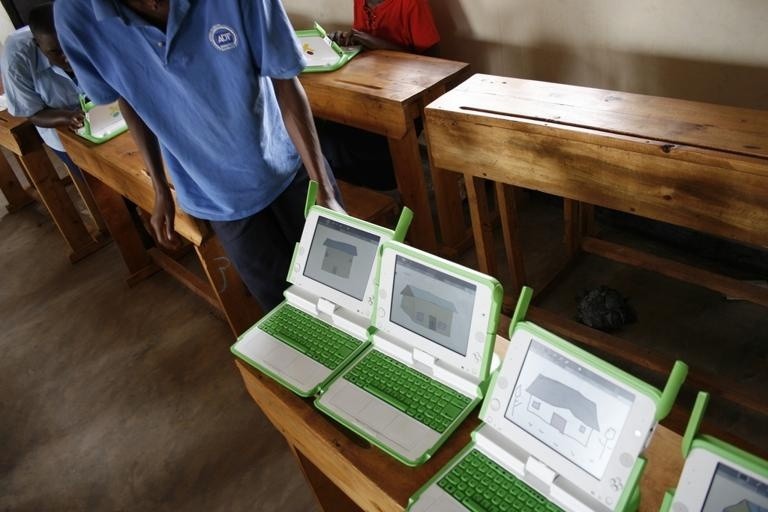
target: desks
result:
[233,308,684,512]
[295,42,521,261]
[423,72,768,414]
[54,108,399,340]
[0,76,116,264]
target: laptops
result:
[291,30,361,73]
[668,436,768,512]
[229,205,414,398]
[69,100,128,145]
[313,241,504,467]
[406,320,688,512]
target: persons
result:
[2,0,85,177]
[49,0,349,325]
